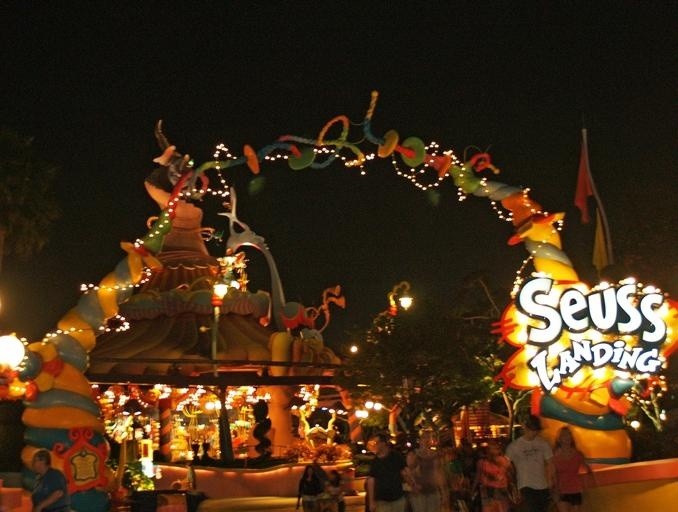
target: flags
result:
[572,139,615,274]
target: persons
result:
[294,425,592,511]
[30,450,67,512]
